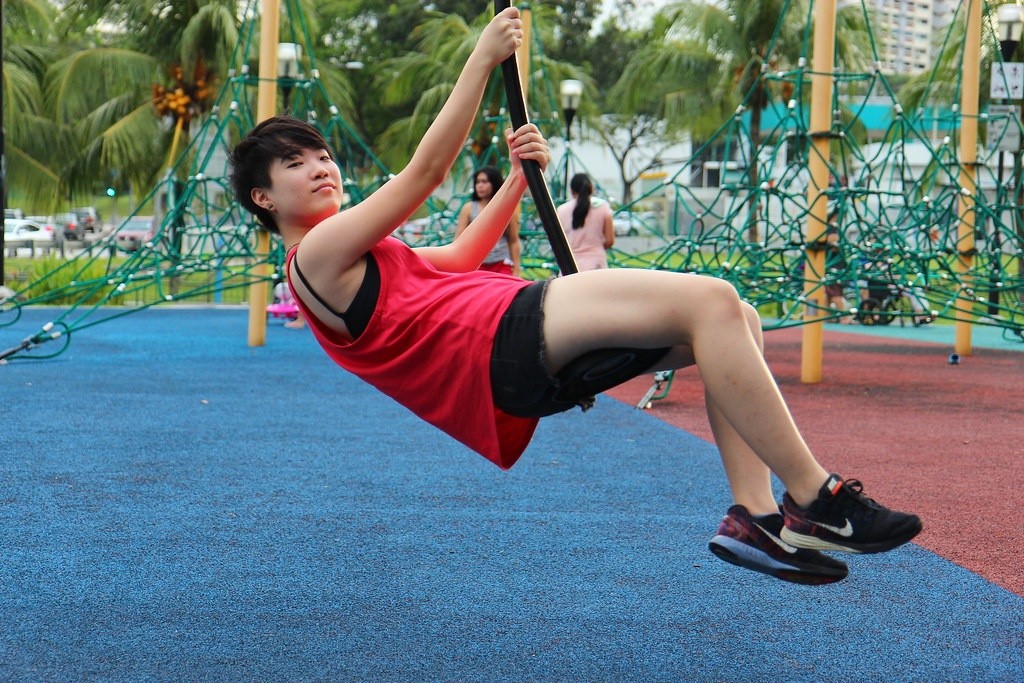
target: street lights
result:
[987,2,1024,316]
[278,42,304,115]
[557,78,580,204]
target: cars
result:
[108,214,155,253]
[611,209,661,236]
[4,218,52,255]
[26,215,64,247]
[52,214,86,240]
[4,208,24,220]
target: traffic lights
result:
[106,187,116,199]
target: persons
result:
[793,222,857,324]
[557,172,614,277]
[452,165,522,278]
[234,6,923,585]
[859,236,936,324]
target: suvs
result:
[72,206,102,233]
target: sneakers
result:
[709,504,848,585]
[780,473,922,554]
